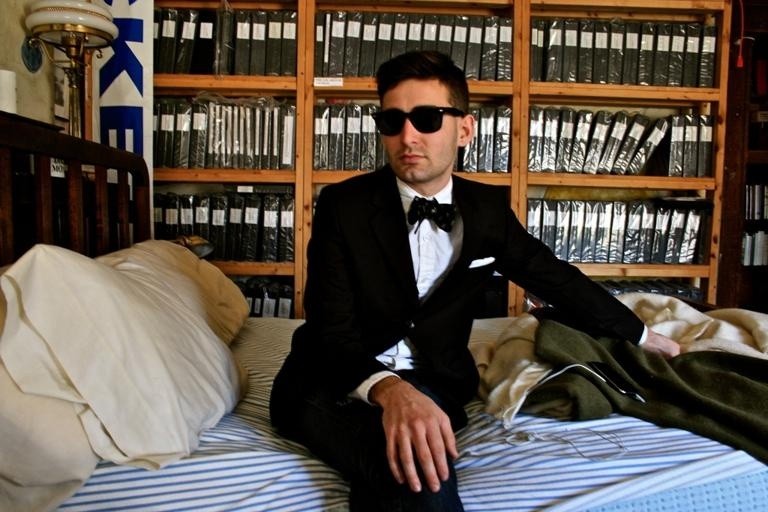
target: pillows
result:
[1,241,249,509]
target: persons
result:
[267,49,683,512]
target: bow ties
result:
[406,195,459,234]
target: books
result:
[741,184,768,267]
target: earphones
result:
[516,430,527,439]
[527,431,545,442]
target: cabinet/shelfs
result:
[302,0,519,321]
[515,0,732,322]
[154,1,306,322]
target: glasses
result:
[370,104,465,137]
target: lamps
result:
[17,2,119,139]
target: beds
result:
[0,108,768,511]
